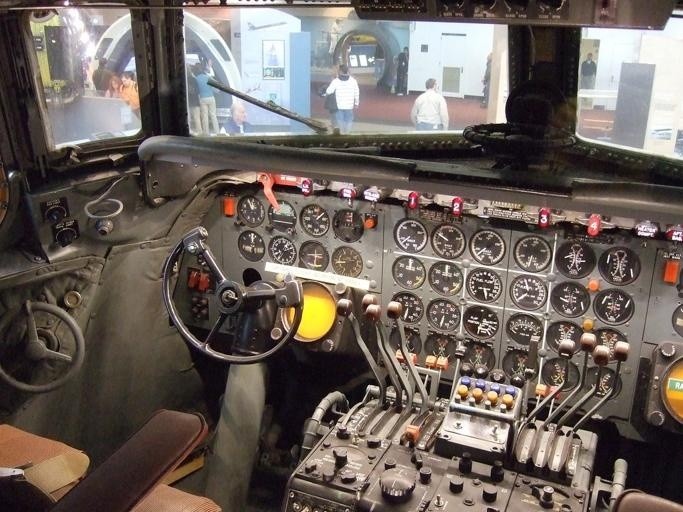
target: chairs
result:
[0,407,220,511]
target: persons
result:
[186,62,201,135]
[324,62,360,134]
[393,44,408,97]
[578,51,596,88]
[219,101,256,134]
[91,57,112,93]
[409,77,450,131]
[317,62,339,128]
[105,74,125,98]
[118,72,141,122]
[193,57,221,135]
[479,51,491,109]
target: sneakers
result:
[397,93,404,97]
